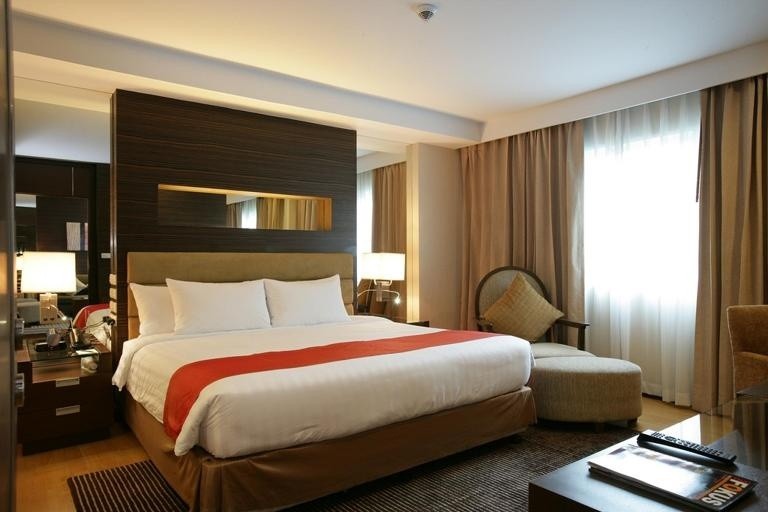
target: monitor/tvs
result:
[14,251,76,294]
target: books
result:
[588,441,759,511]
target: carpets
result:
[67,418,643,511]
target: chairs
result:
[477,266,594,359]
[726,306,768,398]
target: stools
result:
[528,358,645,428]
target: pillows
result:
[130,281,175,338]
[264,274,352,326]
[165,278,271,331]
[66,279,88,296]
[483,274,566,343]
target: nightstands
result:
[16,332,113,456]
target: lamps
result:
[356,253,405,304]
[15,252,76,324]
[72,317,115,343]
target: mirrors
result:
[157,188,333,233]
[16,192,91,326]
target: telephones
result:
[67,319,92,348]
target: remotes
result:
[637,428,738,465]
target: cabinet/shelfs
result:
[528,389,767,512]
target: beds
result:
[112,251,538,509]
[72,304,111,344]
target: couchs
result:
[56,274,89,316]
